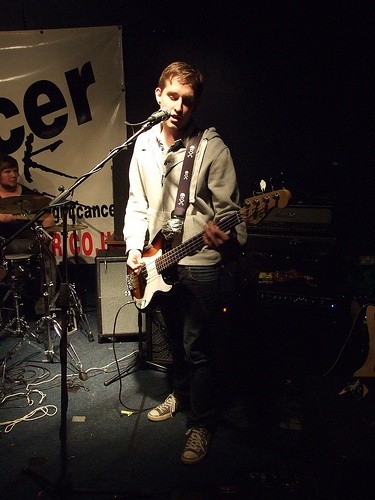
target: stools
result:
[0,253,33,338]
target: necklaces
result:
[17,185,21,196]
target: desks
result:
[104,233,149,249]
[95,249,146,344]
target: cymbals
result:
[0,194,52,215]
[45,224,89,234]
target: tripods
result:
[33,200,95,343]
[1,192,149,380]
[0,262,42,344]
[104,304,170,384]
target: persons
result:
[122,60,249,464]
[0,154,55,239]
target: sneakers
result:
[181,426,211,464]
[148,392,180,421]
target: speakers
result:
[94,256,146,340]
[240,289,350,377]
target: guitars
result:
[125,173,293,314]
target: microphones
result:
[146,109,171,123]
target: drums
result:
[4,254,41,282]
